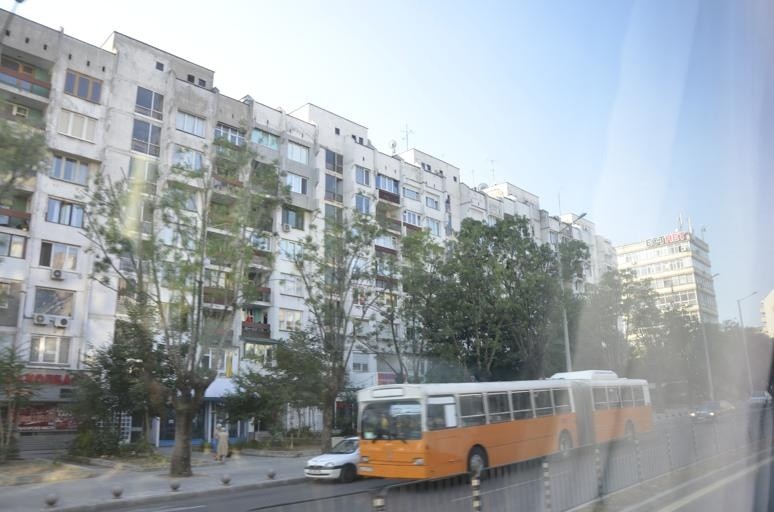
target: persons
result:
[217,427,231,459]
[212,424,222,456]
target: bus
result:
[354,367,654,481]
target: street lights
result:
[553,211,589,372]
[736,291,758,395]
[694,273,721,407]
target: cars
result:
[745,389,773,408]
[688,404,718,423]
[302,435,357,482]
